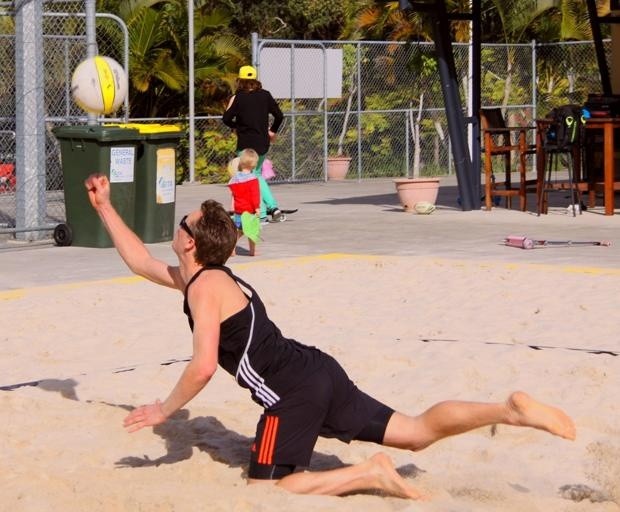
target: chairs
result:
[473,92,619,216]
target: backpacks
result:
[542,104,587,154]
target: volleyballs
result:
[71,56,127,114]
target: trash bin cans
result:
[51,123,186,248]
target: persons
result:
[227,147,260,257]
[221,64,284,221]
[84,169,579,502]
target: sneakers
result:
[259,217,269,224]
[269,212,287,223]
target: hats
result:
[238,65,257,80]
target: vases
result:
[318,156,353,181]
[391,176,443,215]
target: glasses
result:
[178,212,195,240]
